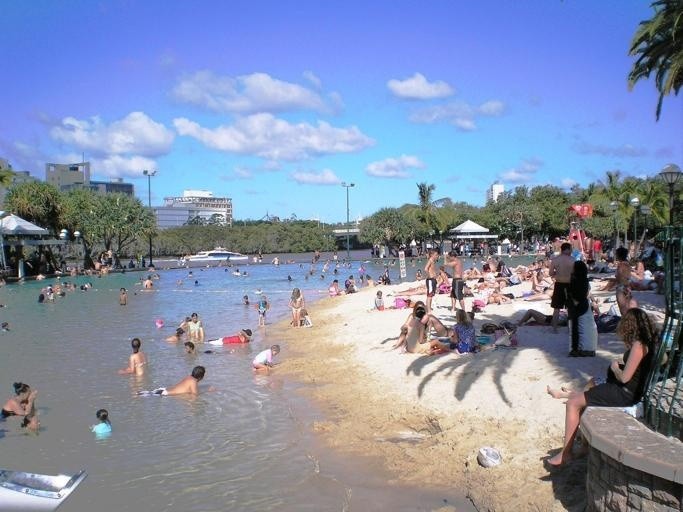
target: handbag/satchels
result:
[495,330,518,345]
[480,323,499,334]
[499,319,515,330]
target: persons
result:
[90,408,112,439]
[20,414,40,432]
[3,381,38,418]
[547,307,669,467]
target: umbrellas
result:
[0,214,49,270]
[449,220,490,235]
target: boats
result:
[180,248,251,263]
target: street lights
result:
[609,199,620,234]
[58,227,69,241]
[140,166,158,268]
[339,180,355,266]
[430,230,444,252]
[656,162,683,319]
[73,230,82,273]
[640,204,651,227]
[630,195,640,243]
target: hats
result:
[656,332,678,351]
